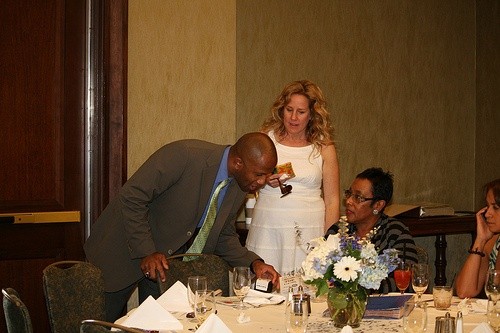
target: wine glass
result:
[410,263,430,308]
[232,266,251,323]
[187,275,216,331]
[393,262,411,295]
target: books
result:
[323,295,415,319]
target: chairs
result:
[0,252,232,332]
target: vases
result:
[327,286,367,329]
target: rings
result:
[146,272,149,277]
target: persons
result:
[85,131,282,330]
[324,167,419,295]
[245,80,340,276]
[454,178,500,299]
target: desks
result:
[377,210,480,288]
[112,289,500,333]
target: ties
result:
[181,181,230,262]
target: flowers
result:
[293,216,403,310]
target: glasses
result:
[344,189,378,203]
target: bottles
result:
[434,311,464,333]
[288,286,312,316]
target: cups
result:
[403,302,427,333]
[284,299,309,333]
[484,269,500,333]
[432,285,454,310]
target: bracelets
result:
[468,247,485,257]
